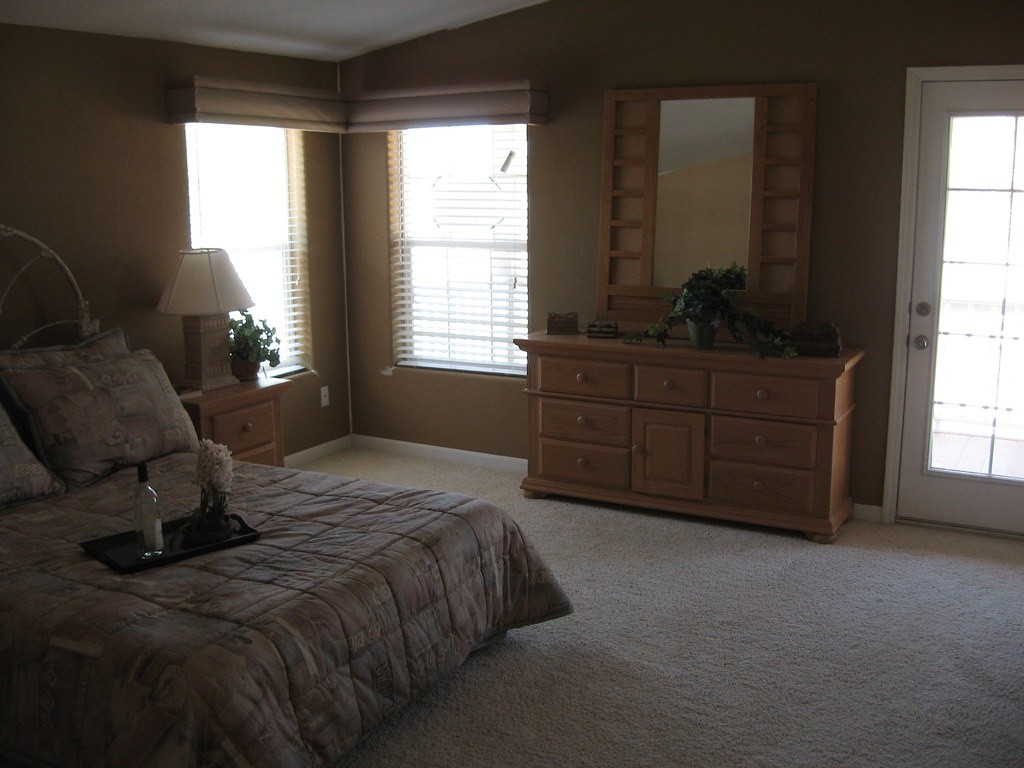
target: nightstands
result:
[177,377,294,467]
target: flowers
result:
[189,438,242,532]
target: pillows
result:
[0,327,129,371]
[0,402,66,509]
[0,348,200,491]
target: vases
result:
[171,520,234,550]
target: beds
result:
[0,223,573,768]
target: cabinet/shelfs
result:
[513,329,867,545]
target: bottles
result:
[134,461,164,559]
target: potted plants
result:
[624,259,799,360]
[229,309,281,382]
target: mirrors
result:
[651,97,756,292]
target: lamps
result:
[155,249,250,392]
